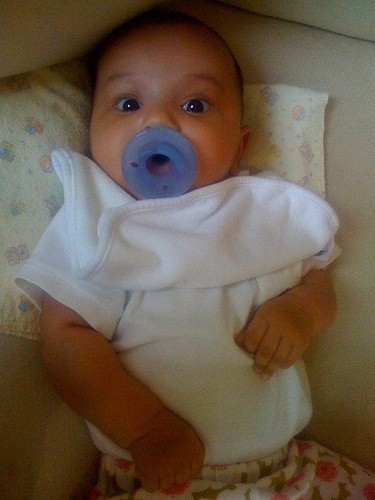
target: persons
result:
[12,7,375,500]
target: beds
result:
[0,0,375,499]
